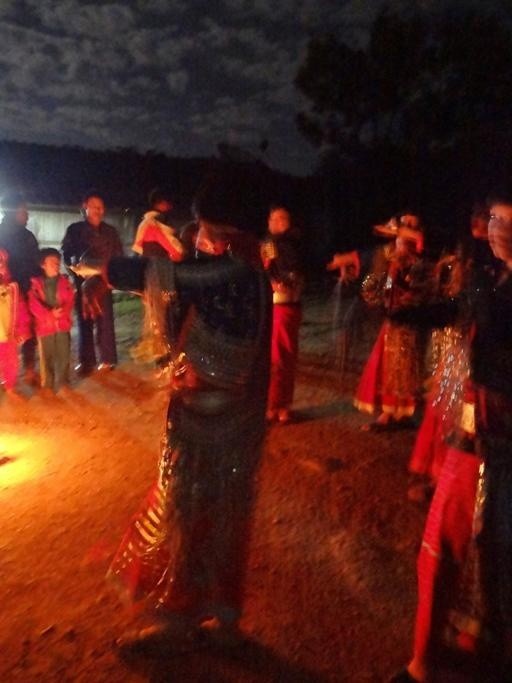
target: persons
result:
[328,213,428,429]
[0,184,199,398]
[258,205,306,425]
[106,181,273,645]
[389,185,512,683]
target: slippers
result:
[358,422,390,433]
[200,625,247,650]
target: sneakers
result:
[117,630,194,659]
[74,363,92,371]
[97,363,114,371]
